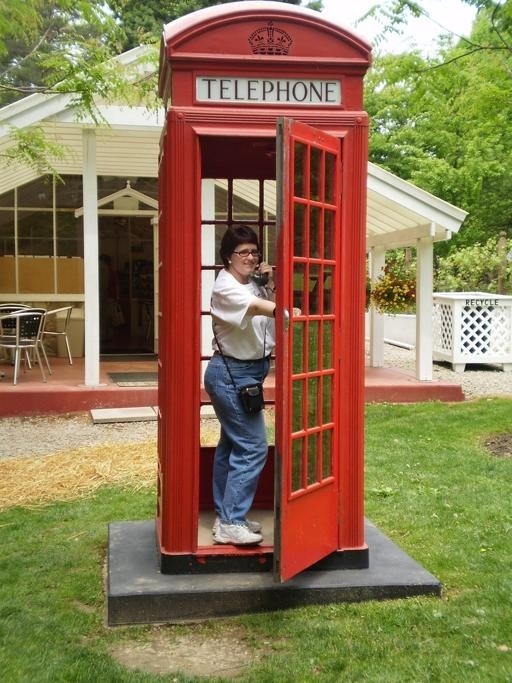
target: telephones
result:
[255,254,269,287]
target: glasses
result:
[233,249,261,258]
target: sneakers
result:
[213,525,263,545]
[211,515,263,534]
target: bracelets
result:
[272,306,277,318]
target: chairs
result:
[0,303,76,386]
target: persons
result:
[98,253,117,345]
[203,220,301,548]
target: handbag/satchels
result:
[239,383,264,414]
[111,304,126,328]
